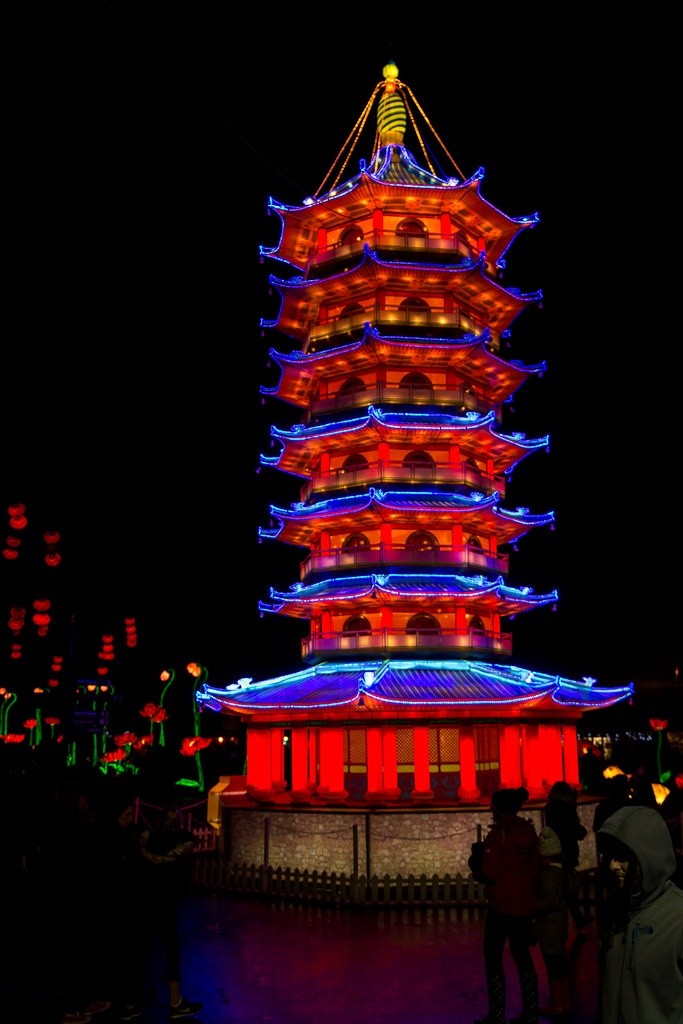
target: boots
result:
[473,976,506,1024]
[510,977,538,1024]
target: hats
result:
[600,840,635,861]
[537,826,561,855]
[492,788,526,816]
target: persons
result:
[468,775,683,1024]
[27,743,202,1024]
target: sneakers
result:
[83,999,111,1015]
[170,997,202,1018]
[63,1011,92,1024]
[121,1005,143,1020]
